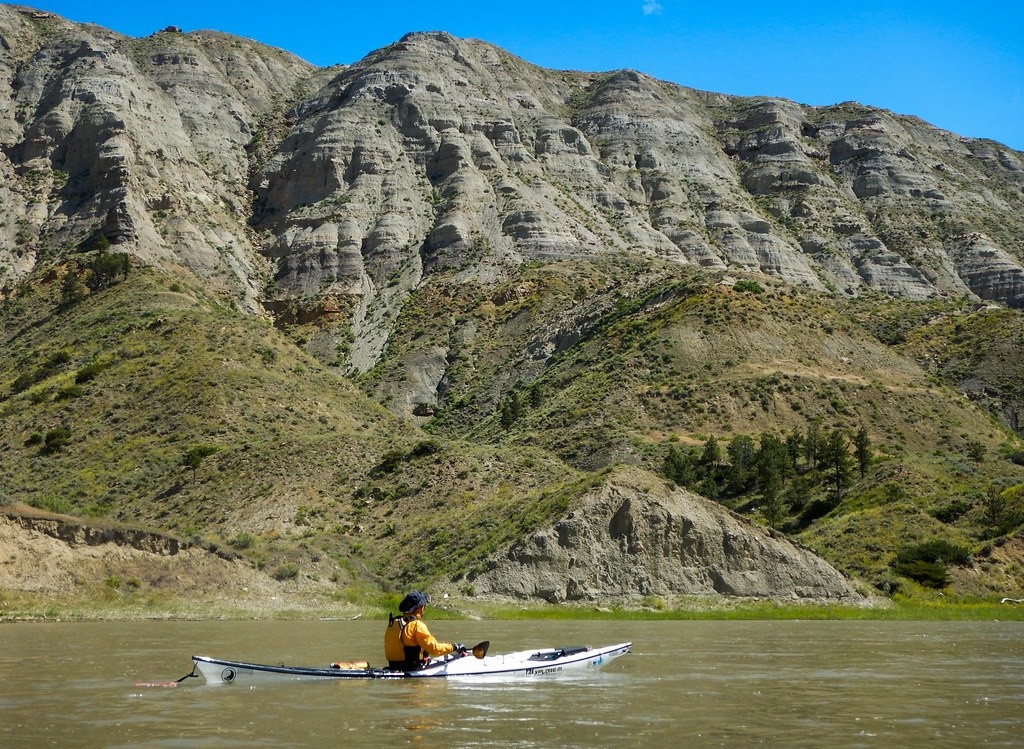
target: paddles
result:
[456,640,490,660]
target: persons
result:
[384,591,469,671]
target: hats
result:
[399,593,426,614]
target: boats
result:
[190,639,633,684]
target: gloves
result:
[454,643,467,654]
